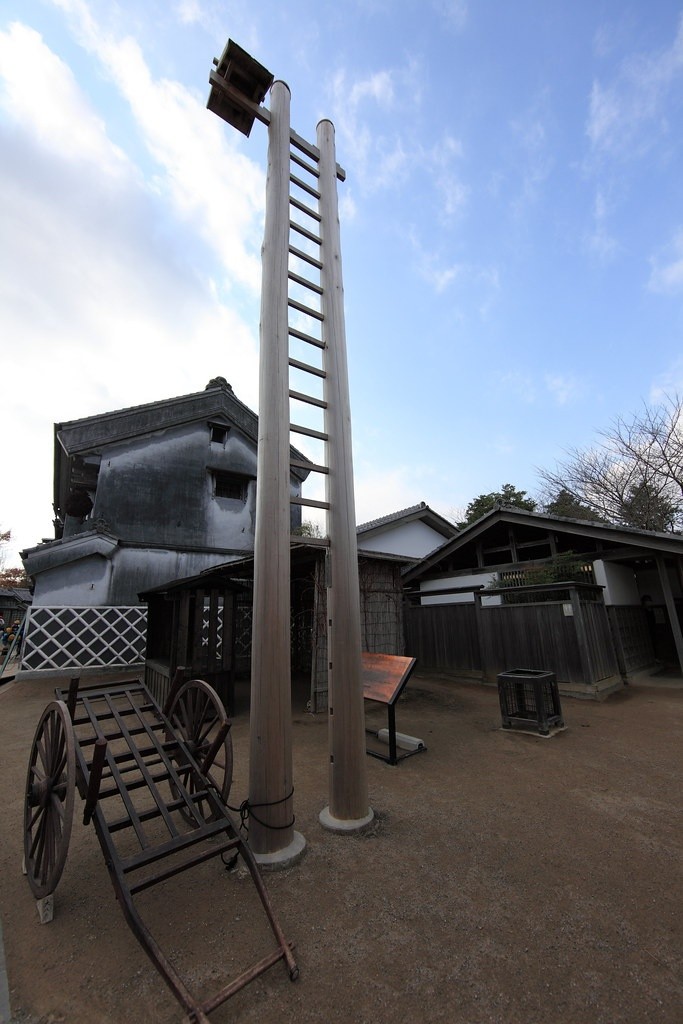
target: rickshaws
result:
[22,673,299,1024]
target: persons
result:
[0,616,5,631]
